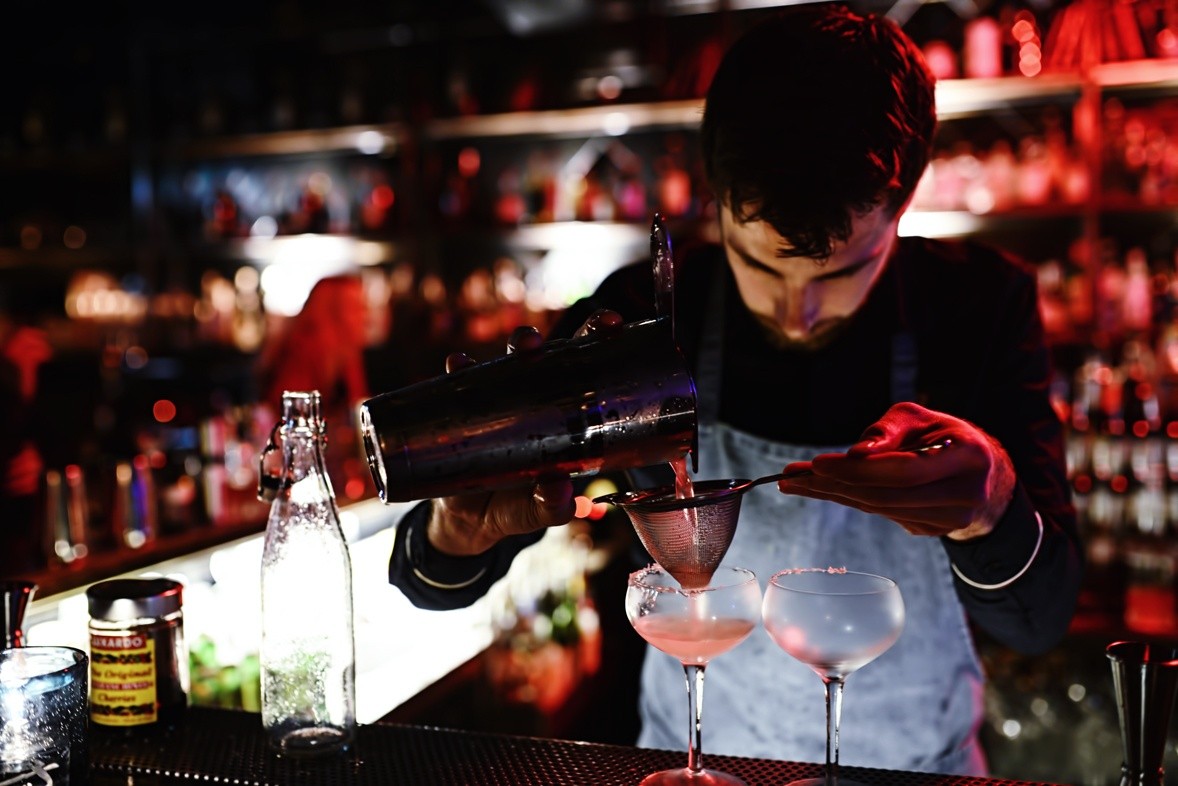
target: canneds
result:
[85,578,185,746]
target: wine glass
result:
[625,563,761,786]
[762,567,906,786]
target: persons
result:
[390,8,1078,782]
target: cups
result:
[360,319,700,501]
[0,645,89,775]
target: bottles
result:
[259,389,359,766]
[1104,637,1178,786]
